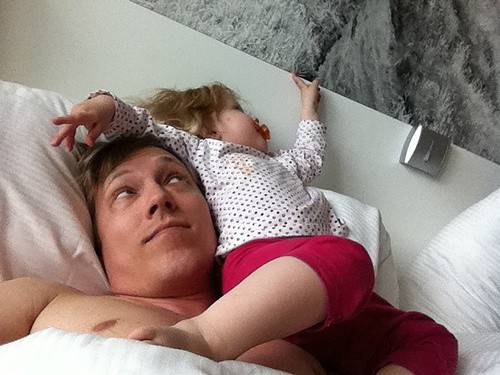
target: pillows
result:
[395,183,500,372]
[2,77,397,336]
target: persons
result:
[0,138,324,375]
[51,73,458,375]
